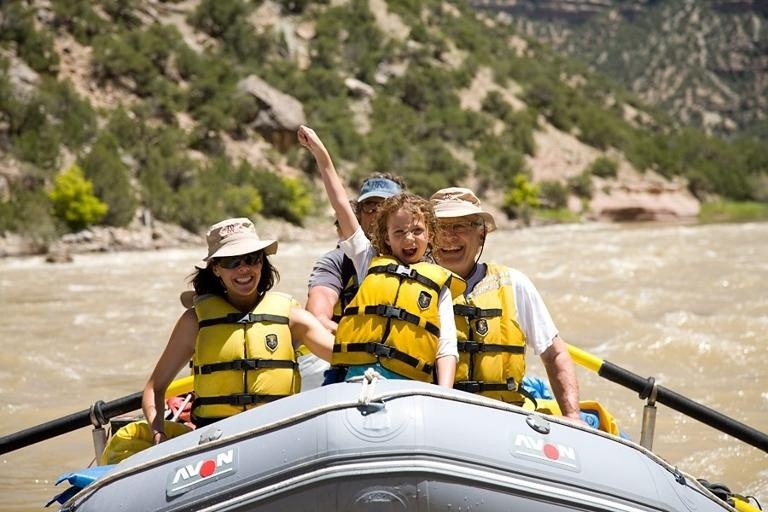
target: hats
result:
[357,177,402,203]
[427,187,497,233]
[194,217,278,270]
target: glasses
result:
[220,252,263,269]
[435,221,480,233]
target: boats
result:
[47,353,765,512]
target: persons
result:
[305,171,406,335]
[142,218,336,445]
[333,199,361,240]
[298,126,468,388]
[424,187,585,426]
